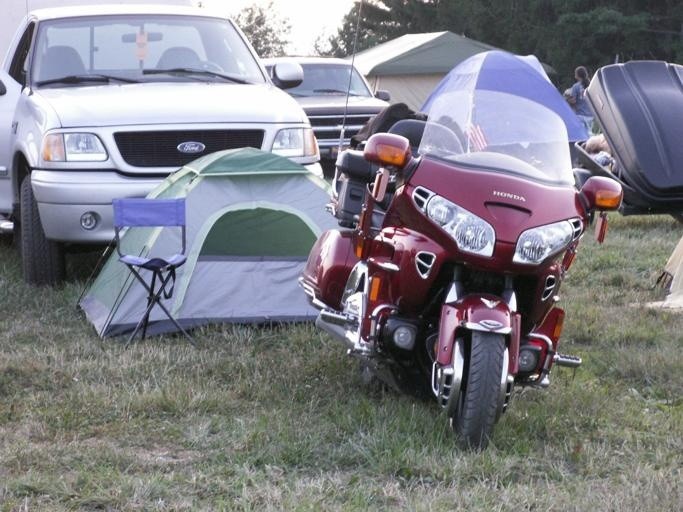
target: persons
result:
[563,65,595,135]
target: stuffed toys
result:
[581,135,618,174]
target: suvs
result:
[250,53,395,176]
[0,5,332,291]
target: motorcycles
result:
[288,45,636,457]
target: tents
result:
[76,147,338,341]
[348,31,588,148]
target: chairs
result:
[113,197,196,350]
[38,46,88,82]
[154,46,200,70]
